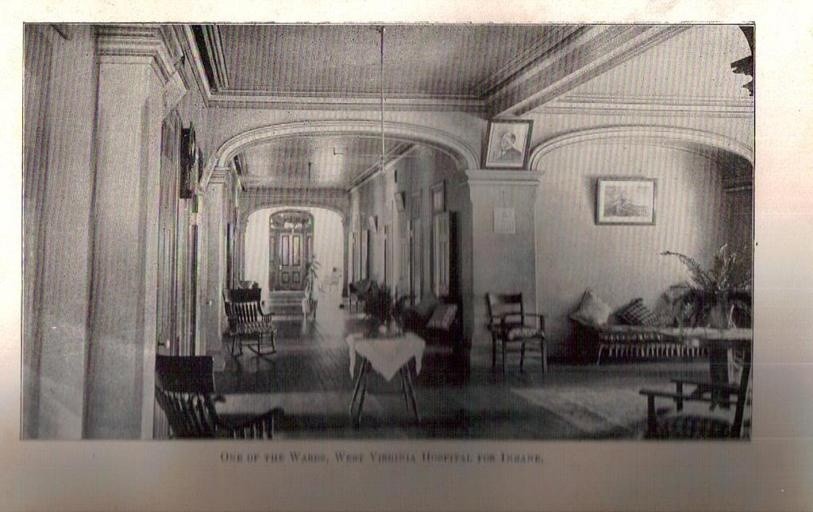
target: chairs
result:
[155,354,284,440]
[222,287,277,358]
[639,351,752,440]
[485,291,548,377]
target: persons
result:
[496,132,523,164]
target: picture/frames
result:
[429,178,445,214]
[596,177,656,226]
[481,118,534,170]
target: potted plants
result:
[657,243,751,344]
[301,254,321,315]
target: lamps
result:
[372,27,397,224]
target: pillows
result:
[568,288,613,328]
[616,298,657,326]
[426,303,458,331]
[416,293,440,317]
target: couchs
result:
[576,318,752,365]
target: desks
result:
[346,330,425,425]
